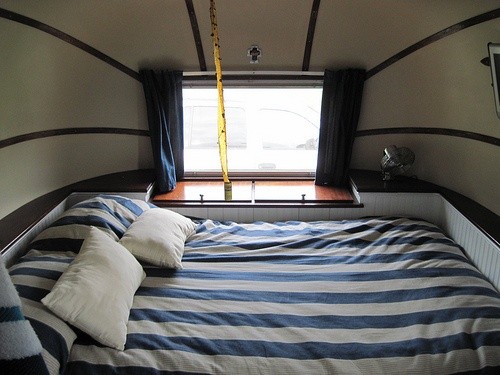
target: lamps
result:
[246,45,262,65]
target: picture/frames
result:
[488,42,500,118]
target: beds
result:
[7,193,500,375]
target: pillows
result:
[41,225,146,352]
[25,193,160,252]
[118,208,198,271]
[7,248,82,375]
